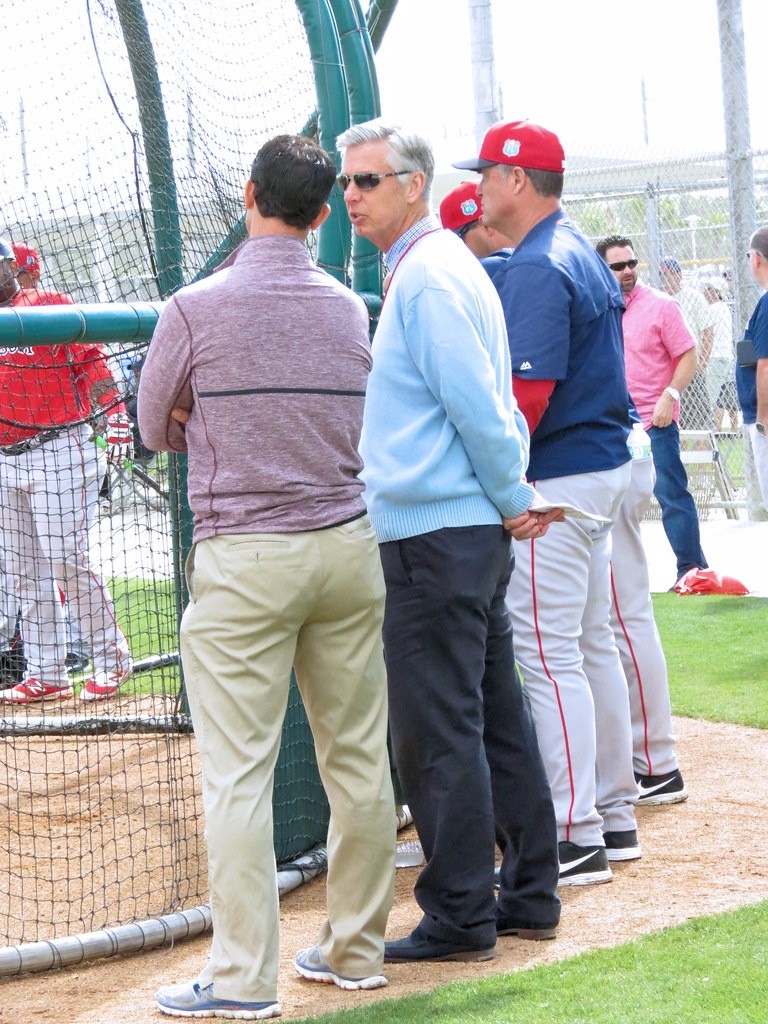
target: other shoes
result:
[64,653,89,674]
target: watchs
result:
[756,422,768,432]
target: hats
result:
[705,276,729,300]
[9,242,40,272]
[451,117,565,173]
[441,181,484,230]
[0,237,16,262]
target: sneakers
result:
[493,841,613,890]
[634,768,688,806]
[79,667,132,700]
[0,671,75,705]
[155,976,282,1020]
[602,830,641,861]
[294,946,389,991]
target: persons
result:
[136,134,398,1020]
[596,235,709,592]
[441,182,688,806]
[452,118,632,888]
[661,256,715,450]
[335,117,564,963]
[0,232,135,704]
[698,276,741,432]
[735,225,768,511]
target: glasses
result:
[335,170,413,191]
[608,259,639,271]
[658,271,676,276]
[746,250,762,259]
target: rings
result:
[536,516,539,524]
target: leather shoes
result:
[495,900,557,941]
[382,924,496,963]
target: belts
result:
[0,429,60,457]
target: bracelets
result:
[665,387,679,400]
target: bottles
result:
[625,423,651,459]
[396,840,424,867]
